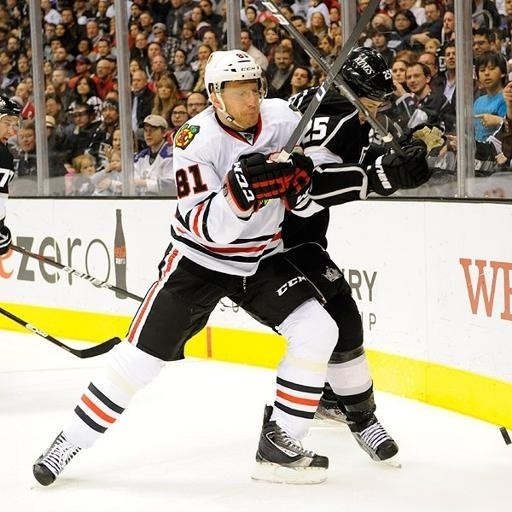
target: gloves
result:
[367,141,430,197]
[282,145,315,213]
[221,154,296,221]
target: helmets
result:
[204,49,265,112]
[0,96,24,129]
[336,47,395,104]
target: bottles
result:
[111,209,132,299]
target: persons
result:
[1,0,511,196]
[0,95,22,279]
[34,48,340,484]
[286,47,429,461]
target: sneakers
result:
[320,382,351,422]
[34,431,83,485]
[256,420,329,467]
[336,411,399,462]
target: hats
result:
[44,116,55,127]
[139,115,167,128]
[99,99,119,114]
[73,53,91,65]
[152,23,167,31]
